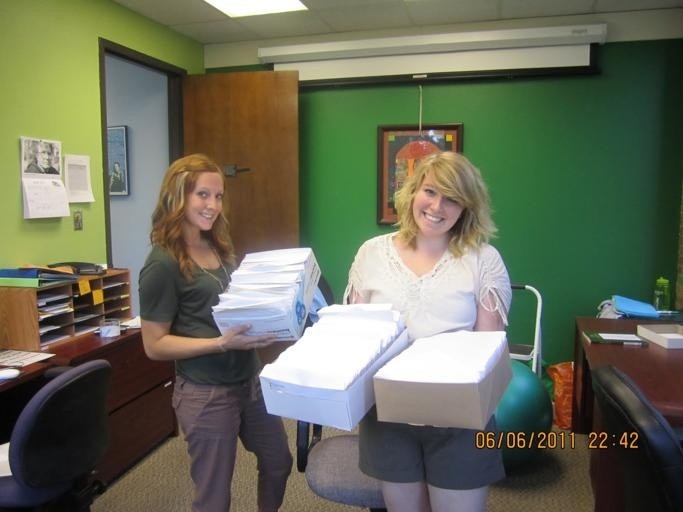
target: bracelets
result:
[217,336,228,352]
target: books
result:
[0,260,107,336]
[582,328,649,347]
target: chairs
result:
[587,362,683,512]
[0,358,113,512]
[296,420,386,512]
[507,285,543,380]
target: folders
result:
[0,268,78,289]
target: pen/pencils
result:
[614,341,648,346]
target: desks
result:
[572,315,683,426]
[0,349,57,393]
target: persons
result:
[24,142,59,174]
[110,161,125,192]
[342,149,514,511]
[136,151,294,511]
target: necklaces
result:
[192,239,230,292]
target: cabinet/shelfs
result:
[1,266,132,351]
[44,324,175,498]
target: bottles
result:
[652,275,672,312]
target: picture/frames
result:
[106,126,129,196]
[376,124,463,224]
